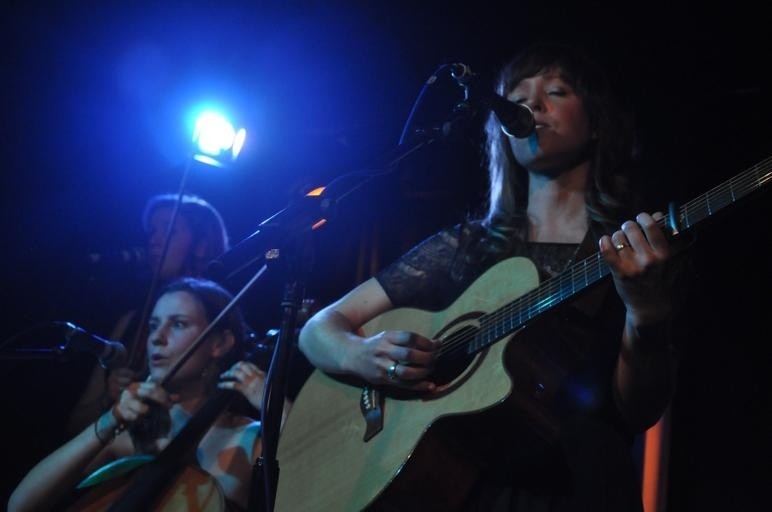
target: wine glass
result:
[614,240,631,255]
[386,362,399,380]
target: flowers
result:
[451,62,534,138]
[51,320,128,370]
[89,247,147,266]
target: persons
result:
[69,194,237,438]
[295,54,691,512]
[8,274,292,510]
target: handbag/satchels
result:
[97,396,110,413]
[110,404,126,433]
[95,418,115,445]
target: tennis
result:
[55,297,323,512]
